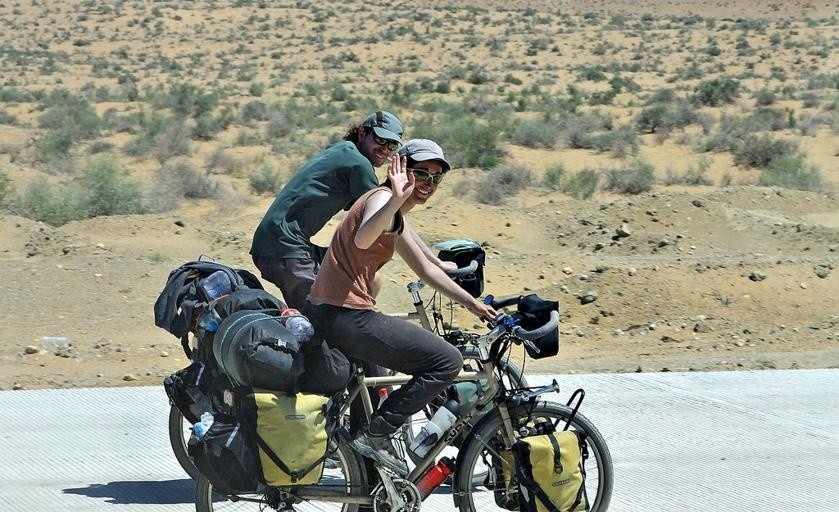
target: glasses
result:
[371,132,399,151]
[406,166,444,185]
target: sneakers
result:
[348,413,409,475]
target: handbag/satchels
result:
[493,450,519,509]
[449,381,494,449]
[518,294,559,359]
[437,243,486,297]
[159,309,355,493]
[518,433,589,511]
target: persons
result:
[247,108,463,473]
[300,136,506,511]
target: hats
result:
[399,138,451,172]
[362,110,404,147]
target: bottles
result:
[278,306,315,342]
[410,398,462,502]
[199,312,222,334]
[377,388,389,410]
[203,269,232,299]
